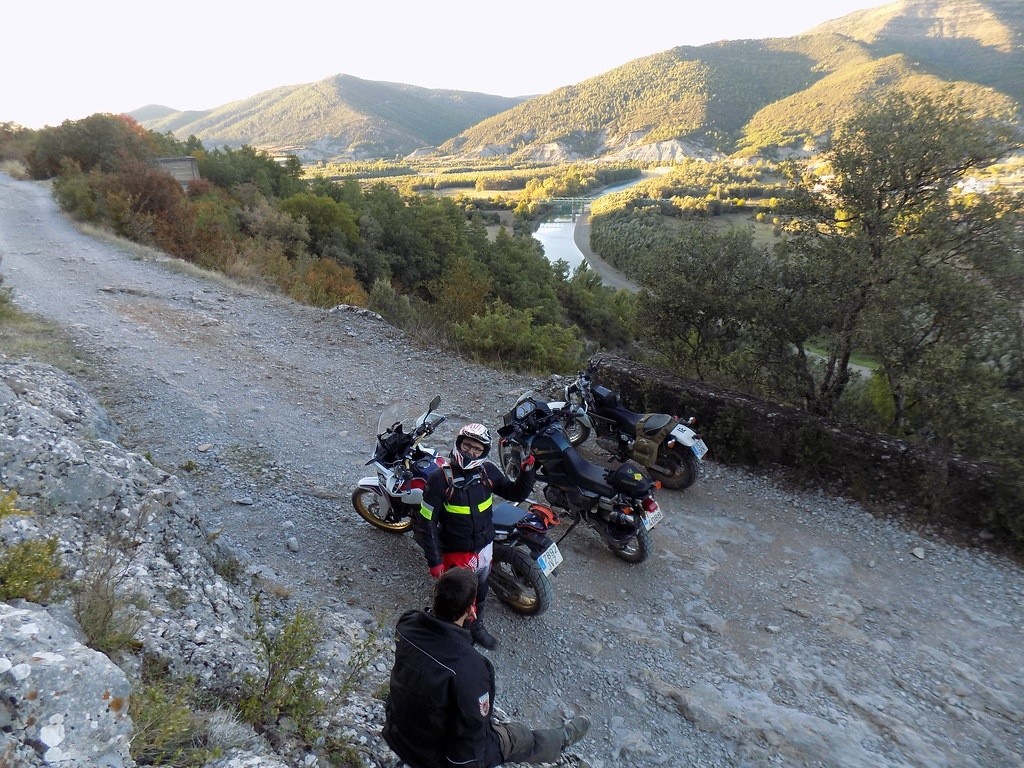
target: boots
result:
[462,618,474,647]
[471,599,498,650]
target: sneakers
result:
[557,715,590,754]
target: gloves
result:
[519,443,535,472]
[430,563,444,578]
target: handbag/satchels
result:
[632,436,659,467]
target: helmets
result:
[451,423,493,471]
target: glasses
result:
[462,442,484,454]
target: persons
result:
[417,424,542,651]
[380,568,591,768]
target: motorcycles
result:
[547,356,709,491]
[495,387,663,565]
[350,394,564,617]
